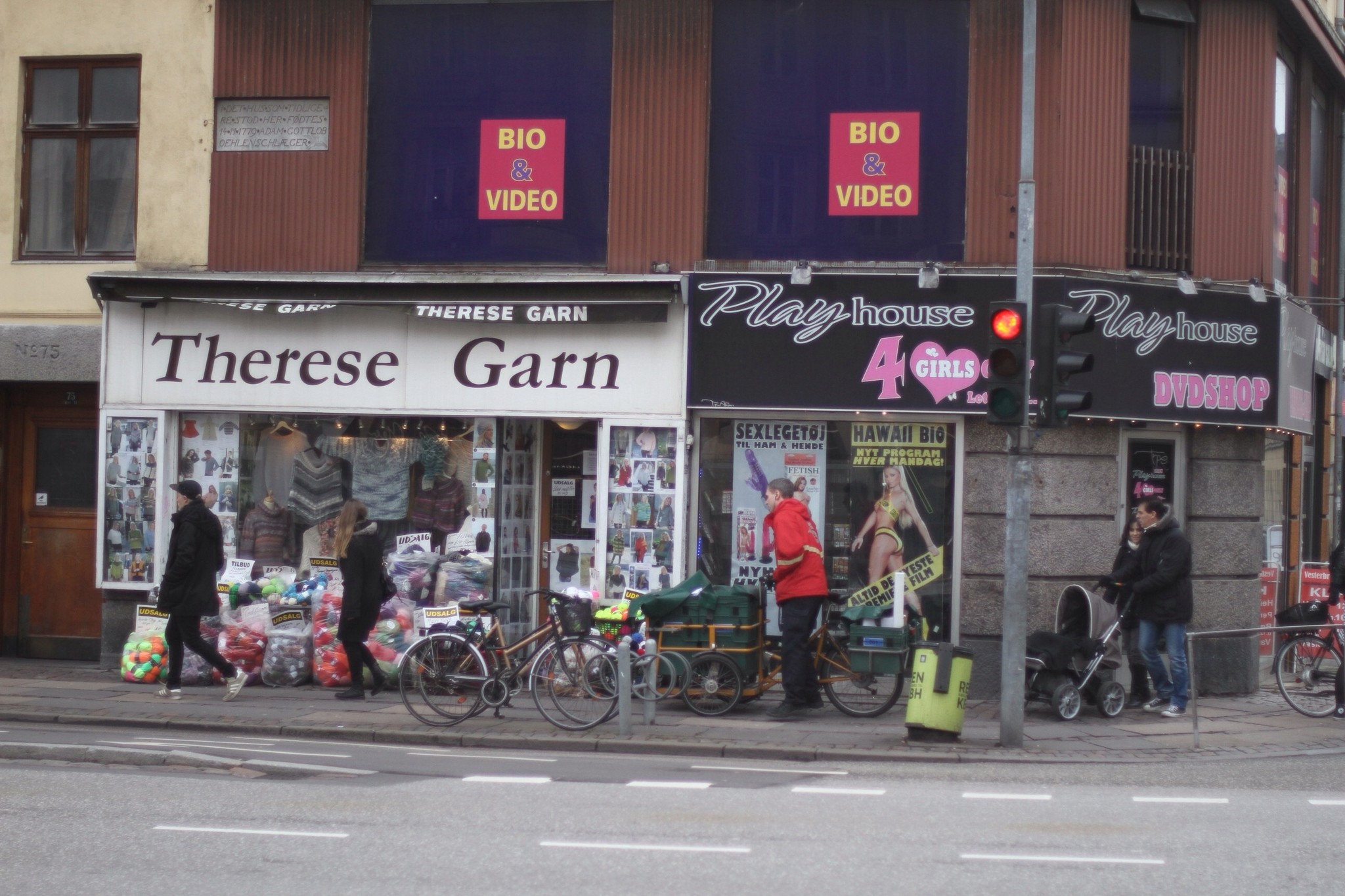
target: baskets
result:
[554,598,592,635]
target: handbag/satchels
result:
[381,555,397,604]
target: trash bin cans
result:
[905,640,974,743]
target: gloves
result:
[1099,576,1114,588]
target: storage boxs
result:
[660,595,766,673]
[850,624,909,673]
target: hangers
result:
[303,445,324,458]
[270,419,293,435]
[425,470,451,479]
[459,424,474,439]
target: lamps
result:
[335,419,343,429]
[1129,270,1199,295]
[292,415,299,427]
[1203,277,1270,302]
[358,418,364,428]
[791,259,821,285]
[402,417,409,429]
[440,417,447,430]
[462,418,468,429]
[416,417,424,430]
[248,414,255,425]
[313,416,321,427]
[379,418,386,428]
[268,414,277,426]
[918,261,946,288]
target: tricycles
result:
[641,575,909,719]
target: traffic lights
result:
[1038,303,1096,430]
[987,301,1026,425]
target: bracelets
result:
[927,543,934,547]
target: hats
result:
[169,480,203,499]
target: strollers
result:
[1026,575,1139,721]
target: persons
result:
[851,463,939,640]
[333,498,390,700]
[240,489,298,580]
[1327,538,1345,721]
[1097,494,1192,717]
[474,421,676,657]
[106,422,234,583]
[765,478,828,716]
[738,477,812,560]
[153,480,248,701]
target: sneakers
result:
[223,668,248,701]
[153,680,181,699]
[1333,703,1345,721]
[1161,705,1187,718]
[1143,697,1170,710]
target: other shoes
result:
[809,698,824,709]
[766,702,808,716]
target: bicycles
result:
[1269,598,1345,718]
[398,587,634,731]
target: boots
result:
[334,675,364,699]
[369,662,392,694]
